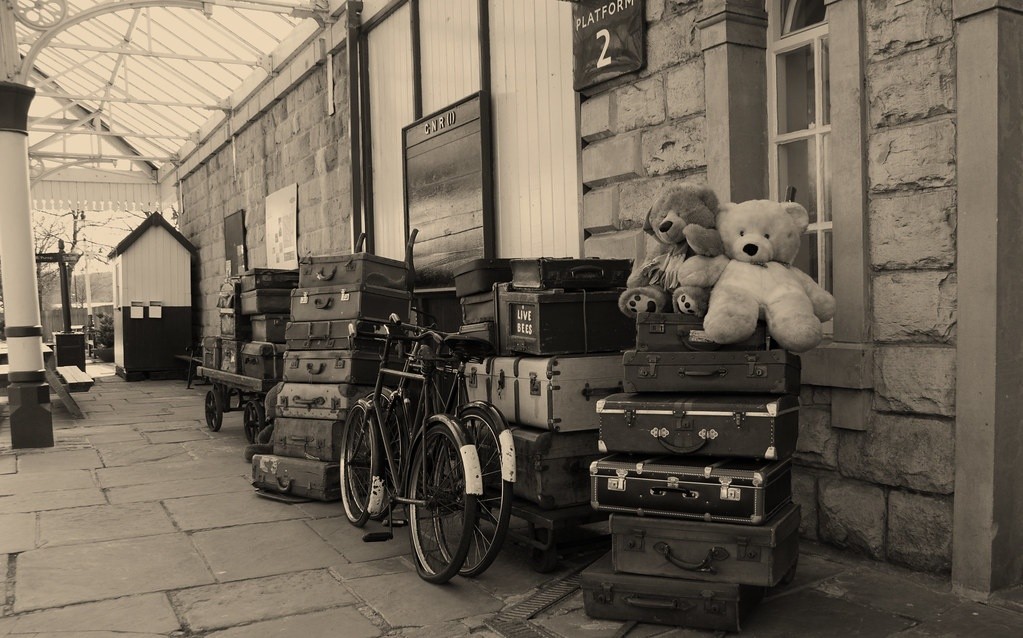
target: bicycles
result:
[354,310,519,578]
[339,322,485,585]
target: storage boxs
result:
[202,252,802,634]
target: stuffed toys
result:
[619,182,720,319]
[675,199,837,350]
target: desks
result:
[0,338,85,419]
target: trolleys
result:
[174,335,286,444]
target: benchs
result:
[56,365,94,392]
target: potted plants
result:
[96,311,115,363]
